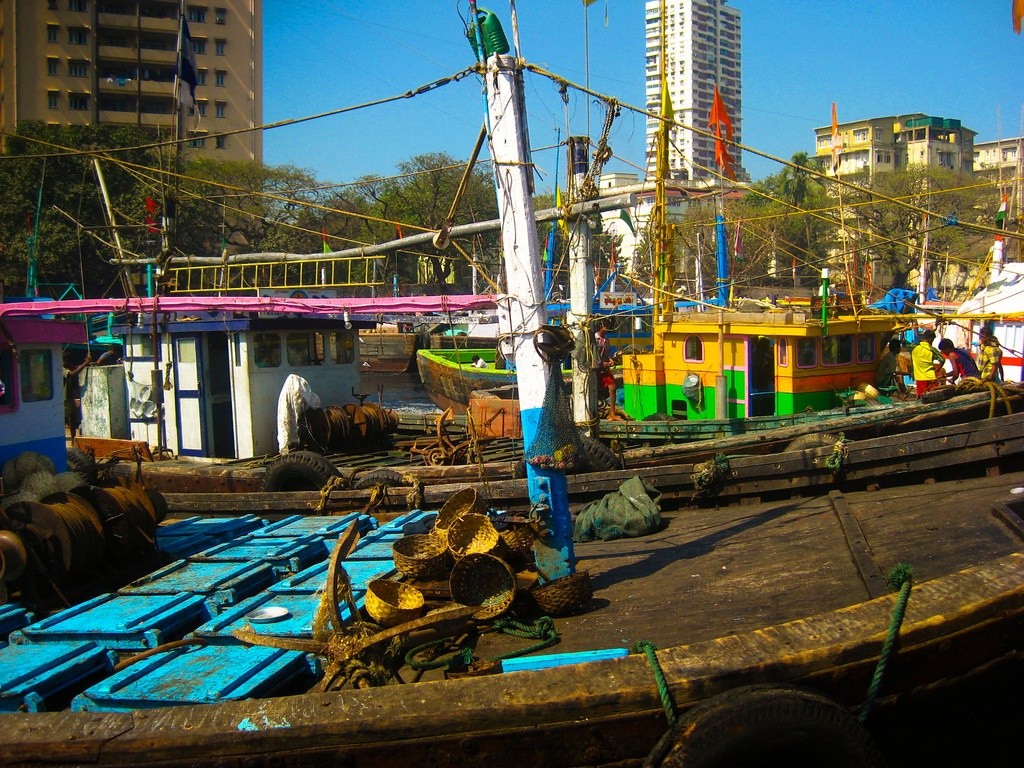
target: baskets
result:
[533,570,594,615]
[500,524,535,558]
[448,514,500,562]
[450,554,518,620]
[435,487,487,533]
[366,578,427,627]
[390,534,449,579]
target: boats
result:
[0,0,1023,768]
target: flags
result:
[995,194,1007,229]
[734,230,744,264]
[832,103,843,168]
[146,198,159,234]
[173,14,201,134]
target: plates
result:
[244,607,289,624]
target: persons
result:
[256,346,274,367]
[471,355,489,369]
[63,352,92,447]
[979,327,1003,379]
[911,330,945,399]
[595,318,624,421]
[96,343,124,365]
[937,338,981,383]
[818,282,848,296]
[877,339,911,399]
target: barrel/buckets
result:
[467,7,509,62]
